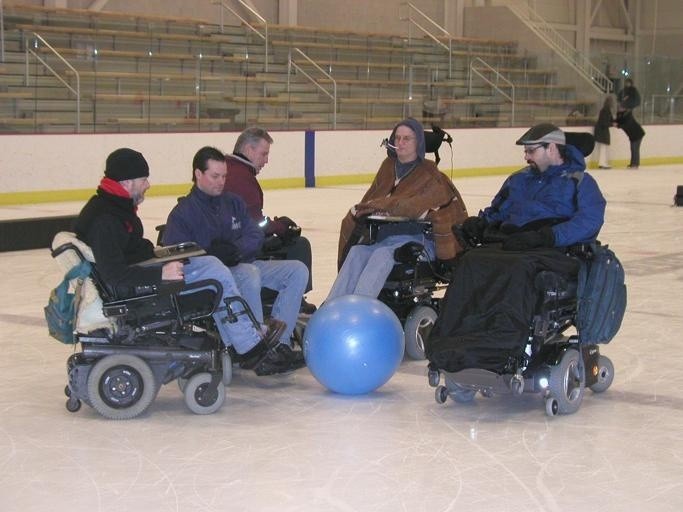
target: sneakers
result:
[300,298,317,314]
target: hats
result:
[516,121,567,147]
[103,147,150,182]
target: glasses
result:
[519,143,545,155]
[392,133,418,142]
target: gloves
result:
[274,214,297,228]
[500,224,556,253]
[208,238,243,267]
[264,218,286,239]
[460,215,489,253]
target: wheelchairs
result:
[154,224,310,376]
[47,232,287,420]
[428,132,613,418]
[340,122,463,360]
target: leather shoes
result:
[238,319,289,371]
[281,344,307,370]
[254,350,293,377]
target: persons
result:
[75,148,280,369]
[324,118,469,303]
[615,103,645,170]
[620,79,642,112]
[429,124,606,373]
[161,145,309,376]
[594,95,615,169]
[223,126,316,314]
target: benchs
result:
[446,37,519,53]
[337,97,483,118]
[290,59,451,82]
[89,93,301,118]
[480,66,557,85]
[247,22,383,45]
[0,67,9,75]
[272,39,434,64]
[0,117,58,134]
[0,92,33,117]
[40,46,257,77]
[4,3,187,34]
[515,98,597,116]
[354,115,510,129]
[494,83,576,100]
[315,77,465,99]
[105,116,328,133]
[469,53,537,68]
[63,70,281,98]
[16,23,232,56]
[530,113,598,126]
